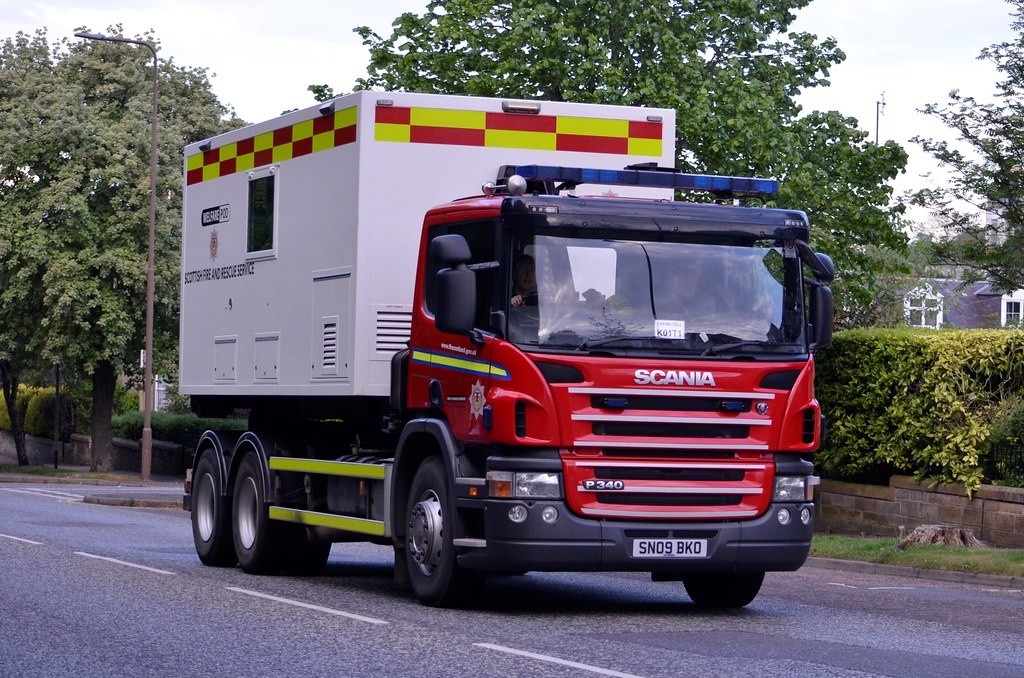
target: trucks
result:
[180,95,837,614]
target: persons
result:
[511,255,553,307]
[637,249,732,314]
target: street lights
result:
[72,32,158,481]
